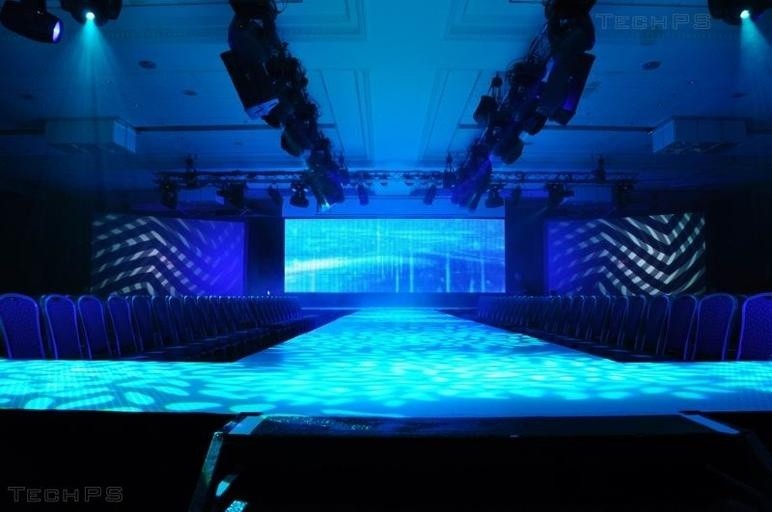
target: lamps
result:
[440,1,596,215]
[611,179,634,211]
[0,1,66,42]
[596,157,609,182]
[219,0,347,213]
[158,174,186,211]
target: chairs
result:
[470,291,772,363]
[0,293,319,358]
